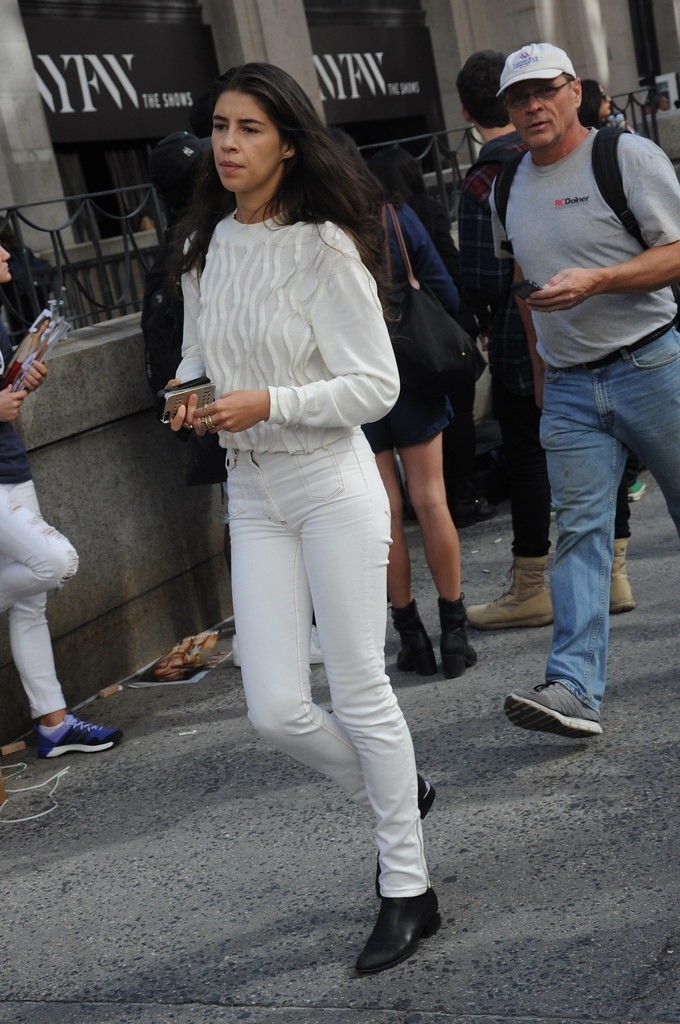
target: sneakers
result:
[33,710,123,758]
[504,682,603,738]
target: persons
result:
[577,78,639,134]
[455,50,635,628]
[0,245,124,759]
[148,130,323,665]
[327,131,477,678]
[133,629,221,683]
[369,150,500,528]
[166,64,442,975]
[2,316,58,391]
[489,42,680,738]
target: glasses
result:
[503,80,572,109]
[602,92,607,100]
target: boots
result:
[438,593,477,678]
[609,537,635,613]
[465,555,554,629]
[387,599,437,676]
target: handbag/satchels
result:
[382,279,477,375]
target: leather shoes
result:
[356,887,440,973]
[375,773,436,898]
[453,497,497,527]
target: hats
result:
[496,42,576,98]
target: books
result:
[2,309,72,391]
[128,629,232,688]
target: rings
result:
[183,423,193,428]
[203,403,208,413]
[201,416,215,431]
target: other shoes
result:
[627,480,646,501]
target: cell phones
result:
[511,279,543,301]
[154,376,216,424]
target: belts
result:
[571,320,674,371]
